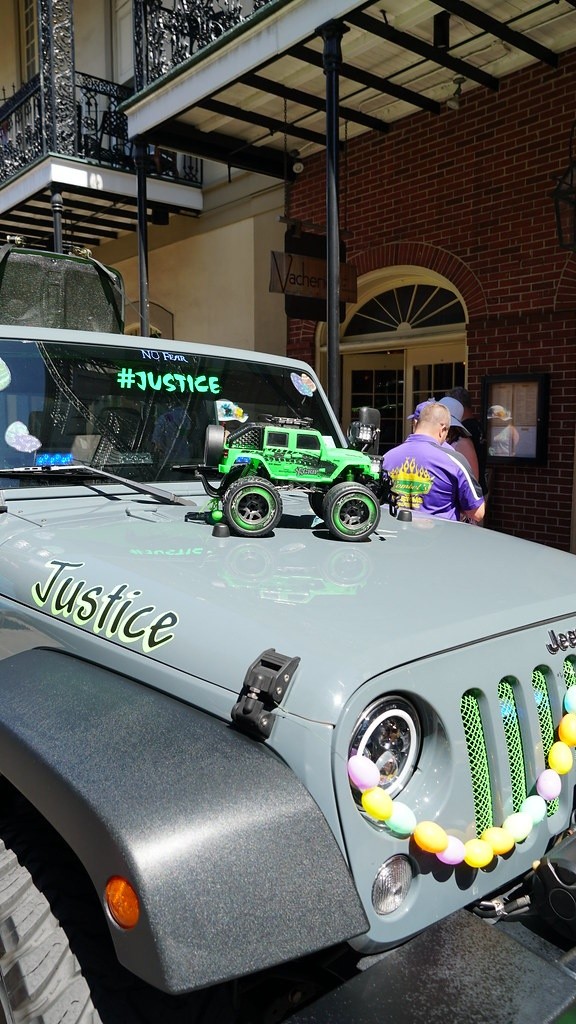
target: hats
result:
[487,405,512,422]
[215,399,249,424]
[407,400,435,420]
[435,397,472,437]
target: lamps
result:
[446,74,466,111]
[290,150,304,173]
[547,120,576,253]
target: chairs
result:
[28,410,87,466]
[95,408,155,457]
[84,111,133,169]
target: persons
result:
[382,386,493,530]
[487,405,518,458]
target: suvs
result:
[194,415,401,544]
[0,323,576,1024]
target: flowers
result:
[496,411,505,417]
[507,410,511,416]
